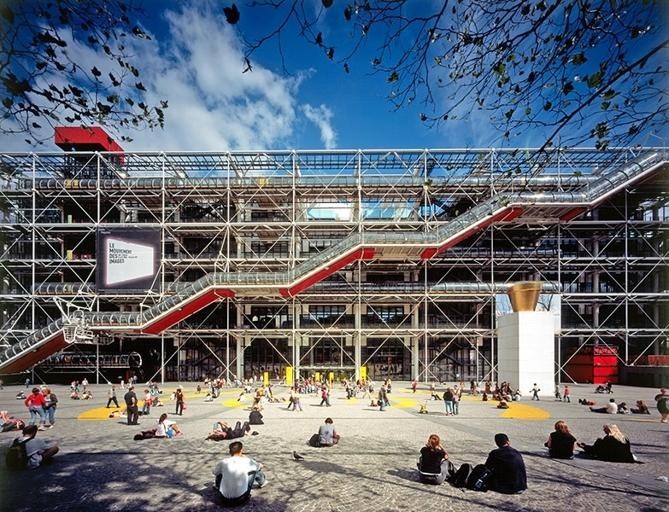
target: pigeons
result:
[293,451,304,461]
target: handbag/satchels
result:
[165,430,175,438]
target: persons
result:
[547,420,578,458]
[483,433,528,495]
[212,441,269,506]
[1,369,669,449]
[576,422,638,463]
[416,435,458,486]
[7,424,61,470]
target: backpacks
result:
[466,462,494,492]
[5,435,33,472]
[447,461,474,489]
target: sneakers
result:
[38,426,46,431]
[212,482,219,490]
[127,421,141,425]
[260,479,270,487]
